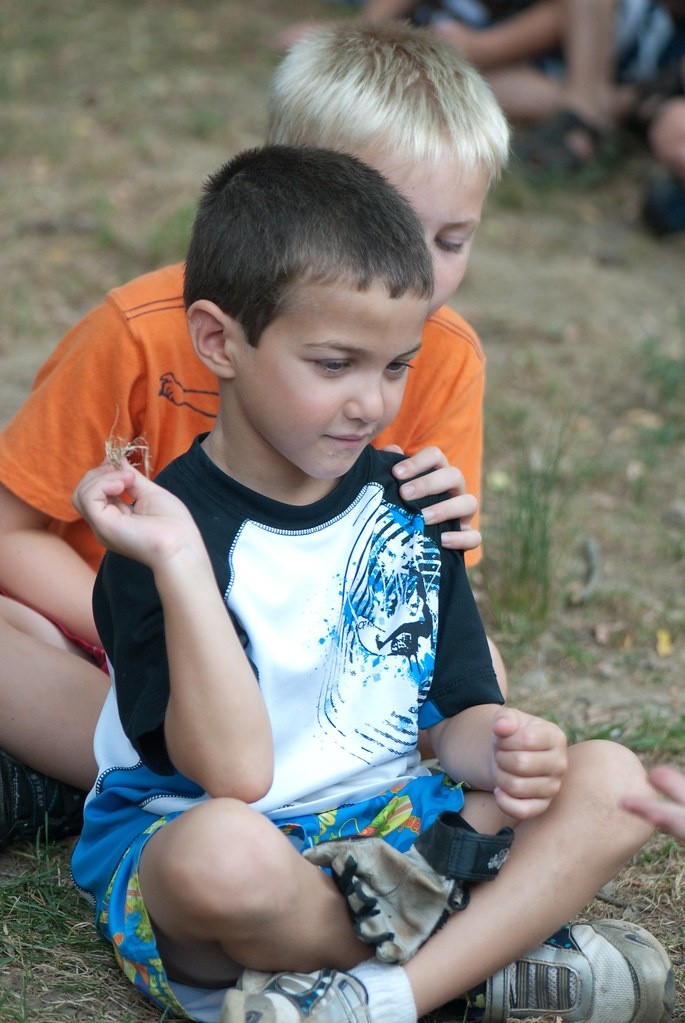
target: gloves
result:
[303,811,514,963]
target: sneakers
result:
[221,967,371,1023]
[453,917,676,1023]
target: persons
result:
[0,19,509,791]
[73,147,675,1023]
[266,0,685,245]
